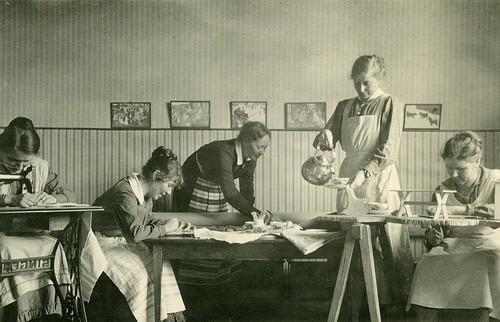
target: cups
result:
[364,202,386,213]
[244,221,255,232]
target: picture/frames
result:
[170,100,210,128]
[403,104,442,130]
[110,102,151,129]
[230,100,267,128]
[284,102,327,130]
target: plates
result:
[327,184,349,190]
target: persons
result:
[312,54,415,305]
[64,144,196,322]
[0,116,76,322]
[169,121,282,287]
[404,130,500,322]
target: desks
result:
[144,235,358,322]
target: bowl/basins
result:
[331,178,349,186]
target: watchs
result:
[364,170,370,179]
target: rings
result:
[40,198,44,202]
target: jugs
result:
[301,146,335,186]
